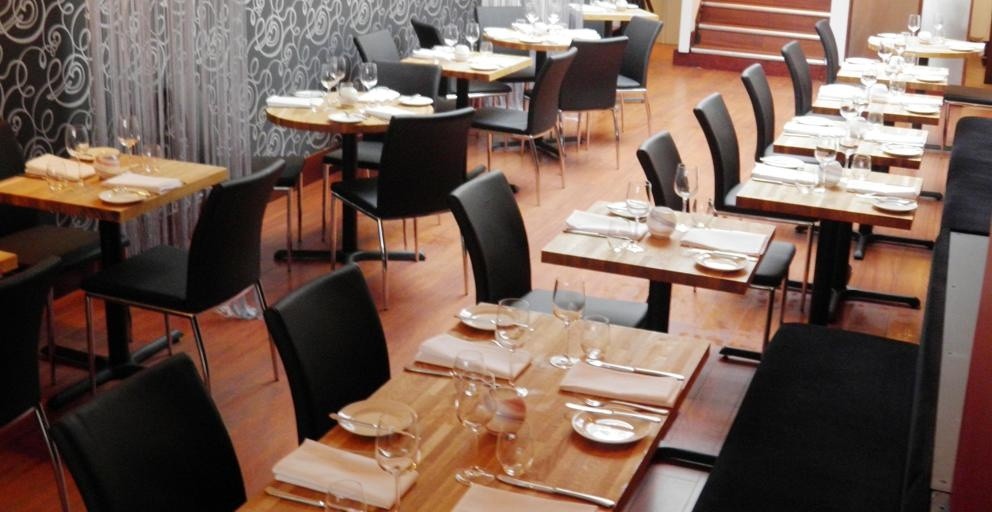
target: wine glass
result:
[372,405,417,510]
[606,161,716,260]
[315,54,378,112]
[547,277,611,372]
[502,0,628,46]
[453,299,540,486]
[814,9,945,193]
[443,21,502,72]
[41,115,164,189]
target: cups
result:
[324,477,369,512]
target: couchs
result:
[691,115,992,512]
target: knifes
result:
[749,177,812,189]
[565,229,631,241]
[586,358,685,381]
[326,413,422,440]
[499,466,617,508]
[261,485,364,512]
[708,249,762,265]
[564,399,661,426]
[401,366,502,389]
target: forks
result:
[585,397,670,416]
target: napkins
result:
[100,172,183,195]
[564,210,648,242]
[450,483,600,512]
[679,227,768,258]
[272,437,418,509]
[266,95,312,109]
[413,333,534,382]
[24,153,97,183]
[556,361,680,408]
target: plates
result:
[397,93,437,105]
[761,152,810,171]
[869,192,923,217]
[295,87,328,100]
[947,40,978,54]
[694,251,749,276]
[573,409,653,445]
[97,189,151,204]
[336,399,412,438]
[327,111,368,126]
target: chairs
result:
[446,167,649,329]
[1,254,64,432]
[352,1,663,209]
[636,131,796,351]
[330,105,477,313]
[693,93,820,313]
[37,330,249,511]
[265,261,391,446]
[76,156,287,397]
